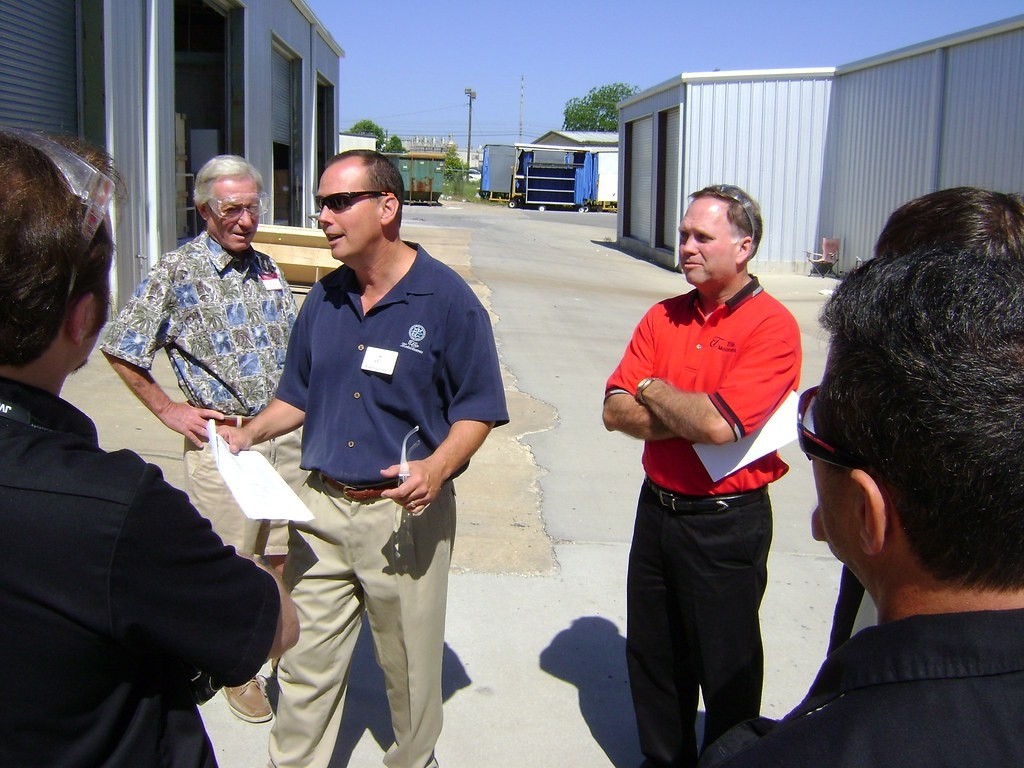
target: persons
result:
[210,151,510,767]
[830,187,1024,649]
[707,251,1024,768]
[101,156,315,723]
[0,125,301,768]
[602,188,802,768]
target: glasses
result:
[315,188,388,210]
[206,190,270,220]
[712,184,756,244]
[0,124,115,303]
[797,386,882,481]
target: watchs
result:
[637,377,660,405]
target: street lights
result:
[464,88,477,181]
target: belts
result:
[319,472,400,502]
[215,417,252,428]
[644,474,768,513]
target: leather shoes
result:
[222,675,274,723]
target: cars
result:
[461,168,482,183]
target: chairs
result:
[803,236,840,279]
